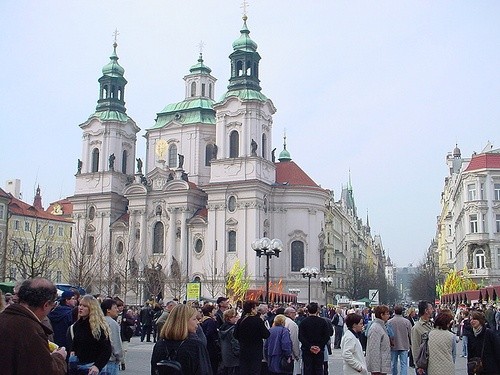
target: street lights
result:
[320,276,333,307]
[251,237,283,306]
[300,266,318,304]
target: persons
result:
[136,158,143,173]
[272,148,277,164]
[116,299,160,344]
[466,314,500,375]
[101,299,126,375]
[75,159,83,175]
[153,300,500,357]
[177,154,184,167]
[211,143,218,160]
[250,139,258,156]
[341,301,458,375]
[109,154,115,171]
[66,294,113,375]
[150,302,332,375]
[0,277,67,375]
[0,280,112,346]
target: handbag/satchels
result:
[280,357,293,371]
[416,333,428,369]
[467,357,484,375]
[123,309,134,338]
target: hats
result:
[61,290,75,300]
[493,304,496,307]
[217,297,228,304]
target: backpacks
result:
[155,338,185,375]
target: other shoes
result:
[459,355,467,358]
[334,347,341,349]
[329,352,332,355]
[147,341,151,342]
[409,364,415,368]
[141,341,143,342]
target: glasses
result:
[49,303,54,312]
[384,313,390,316]
